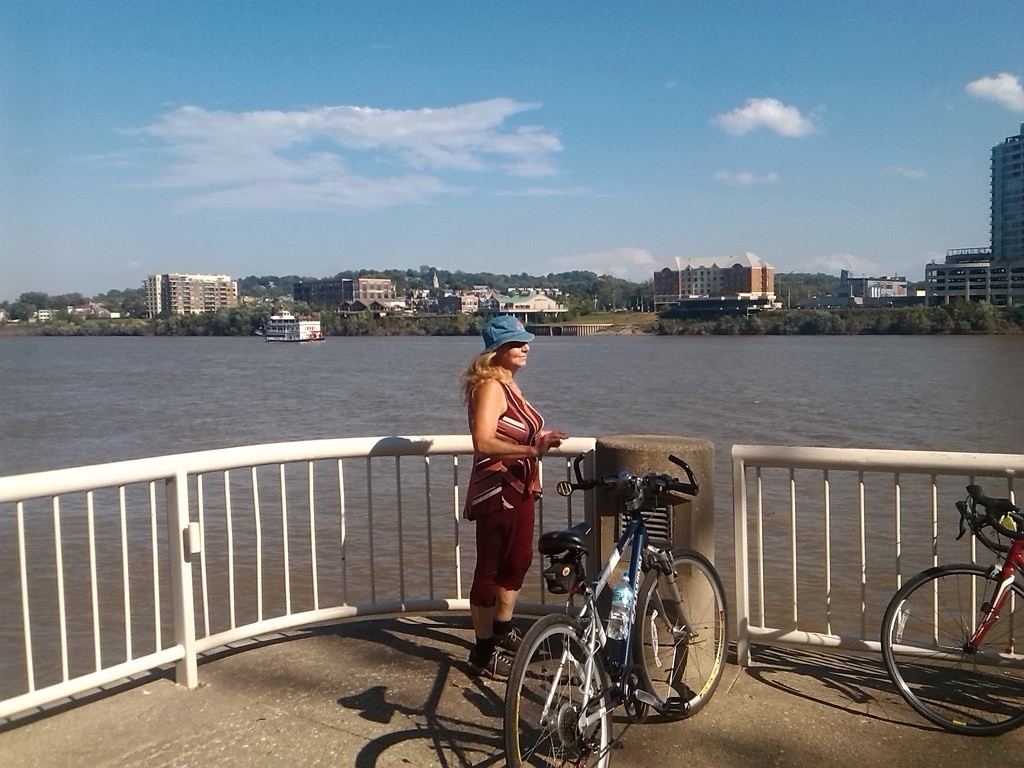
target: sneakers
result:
[469,648,514,681]
[492,628,541,657]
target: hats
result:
[479,314,535,356]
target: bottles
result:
[605,572,634,642]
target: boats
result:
[264,305,325,342]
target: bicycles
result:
[881,485,1024,736]
[499,447,730,768]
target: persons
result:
[464,316,568,682]
